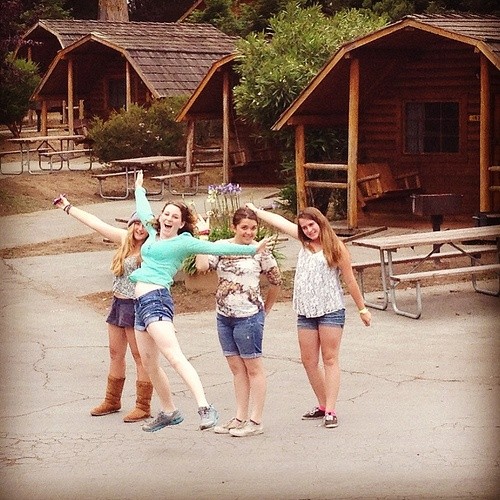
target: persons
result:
[128,170,275,432]
[53,194,155,421]
[195,207,284,437]
[245,203,372,428]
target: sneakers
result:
[230,422,264,436]
[302,406,325,419]
[214,417,240,433]
[198,405,219,430]
[324,410,339,427]
[141,409,183,432]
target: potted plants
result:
[183,253,219,291]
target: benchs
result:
[90,170,148,179]
[357,162,423,220]
[390,264,500,282]
[352,248,497,269]
[150,170,205,180]
[0,148,51,156]
[38,149,94,156]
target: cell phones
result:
[53,194,66,205]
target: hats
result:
[127,209,155,228]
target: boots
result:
[90,375,125,415]
[124,380,152,422]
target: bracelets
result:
[359,308,368,314]
[63,204,72,215]
[199,230,209,235]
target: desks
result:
[91,155,206,201]
[0,135,92,175]
[350,225,500,319]
